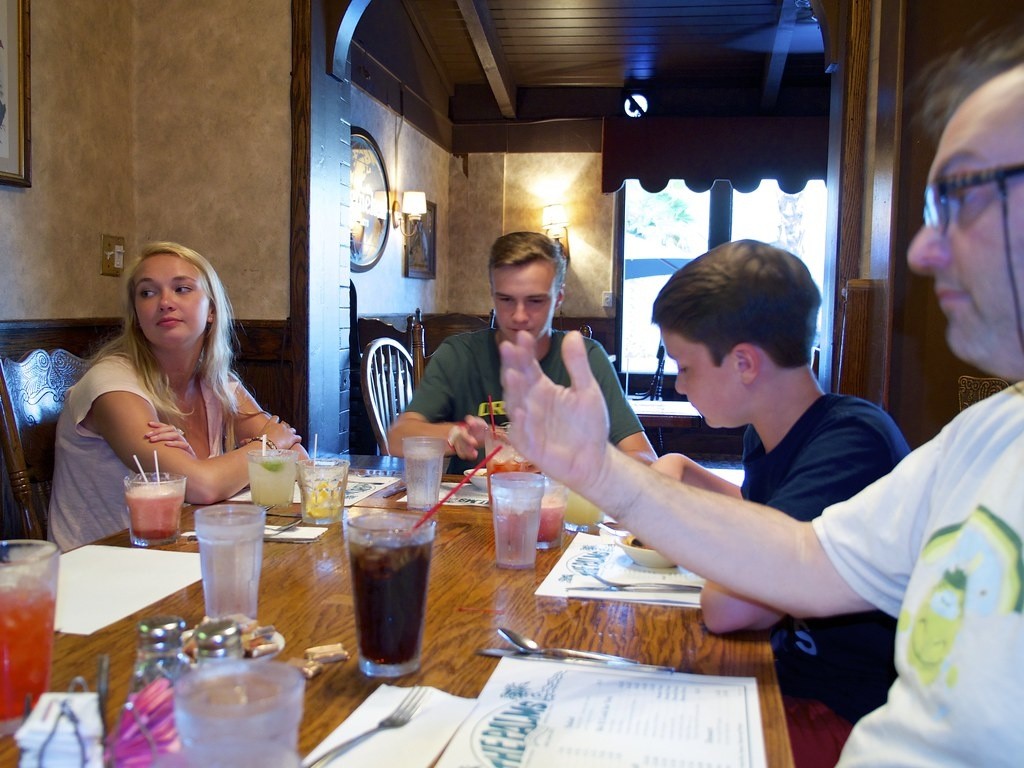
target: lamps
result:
[611,77,650,121]
[391,189,426,236]
[540,202,570,264]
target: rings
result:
[177,428,185,435]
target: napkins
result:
[301,684,478,768]
[181,525,329,540]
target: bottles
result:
[128,615,192,690]
[195,621,246,661]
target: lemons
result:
[260,460,288,473]
[306,482,338,518]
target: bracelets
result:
[447,422,466,453]
[249,436,277,449]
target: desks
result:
[627,399,703,428]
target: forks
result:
[304,684,427,768]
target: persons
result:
[48,244,313,555]
[650,239,911,768]
[389,231,659,475]
[500,18,1024,768]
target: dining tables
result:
[0,467,797,768]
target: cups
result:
[122,471,188,547]
[564,486,603,532]
[484,427,529,508]
[537,477,566,550]
[489,472,545,570]
[246,448,299,507]
[195,504,265,620]
[402,435,448,512]
[0,539,60,734]
[172,658,302,768]
[346,513,436,677]
[296,459,348,526]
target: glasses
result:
[923,164,1024,235]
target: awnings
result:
[601,116,829,194]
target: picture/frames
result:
[403,201,438,279]
[351,125,391,273]
[0,0,31,188]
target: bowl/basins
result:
[615,535,678,568]
[465,469,488,491]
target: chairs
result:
[351,309,495,458]
[0,347,93,539]
[623,341,666,454]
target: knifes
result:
[472,649,676,673]
[383,486,405,498]
[564,586,699,594]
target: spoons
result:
[498,627,642,665]
[265,520,302,533]
[589,573,701,591]
